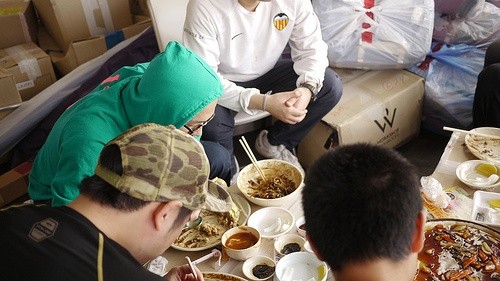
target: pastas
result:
[247,173,297,199]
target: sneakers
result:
[230,155,240,186]
[255,130,305,179]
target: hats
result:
[95,123,233,213]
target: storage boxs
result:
[431,131,479,193]
[297,66,425,171]
[0,0,152,121]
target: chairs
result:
[147,0,274,137]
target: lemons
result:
[475,162,497,177]
[316,265,326,281]
[488,199,500,209]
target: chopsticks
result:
[239,136,267,183]
[443,126,500,139]
[184,255,199,281]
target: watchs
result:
[300,83,318,101]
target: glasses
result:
[183,113,215,135]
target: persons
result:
[27,40,236,209]
[0,123,210,281]
[301,141,426,281]
[183,0,344,181]
[473,39,500,131]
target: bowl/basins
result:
[222,226,260,261]
[470,190,500,228]
[414,218,500,281]
[274,252,328,281]
[304,240,315,254]
[237,159,304,206]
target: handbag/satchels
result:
[312,0,500,138]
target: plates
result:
[274,235,306,255]
[242,256,276,281]
[455,159,500,190]
[464,126,500,160]
[247,206,292,238]
[171,191,252,251]
[201,272,248,281]
[296,216,305,237]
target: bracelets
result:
[261,90,273,112]
